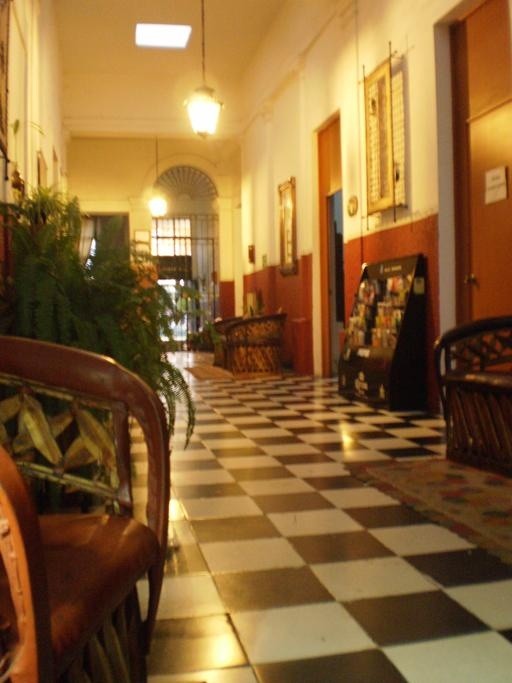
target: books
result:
[346,273,410,346]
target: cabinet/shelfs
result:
[334,252,429,412]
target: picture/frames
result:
[277,176,299,276]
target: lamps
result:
[182,0,227,141]
[145,136,168,219]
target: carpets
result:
[183,364,231,379]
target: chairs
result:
[431,313,511,477]
[209,312,290,380]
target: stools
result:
[0,333,181,683]
